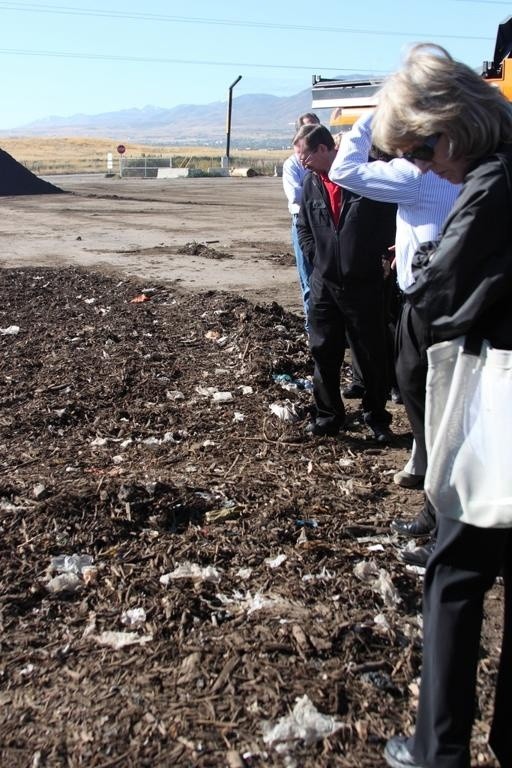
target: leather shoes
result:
[402,543,434,566]
[386,740,420,767]
[392,518,431,537]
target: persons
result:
[366,38,512,768]
[281,114,472,564]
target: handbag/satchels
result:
[423,336,512,529]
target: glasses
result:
[402,132,439,164]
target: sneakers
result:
[307,421,338,433]
[343,385,364,398]
[396,467,422,486]
[373,425,394,444]
